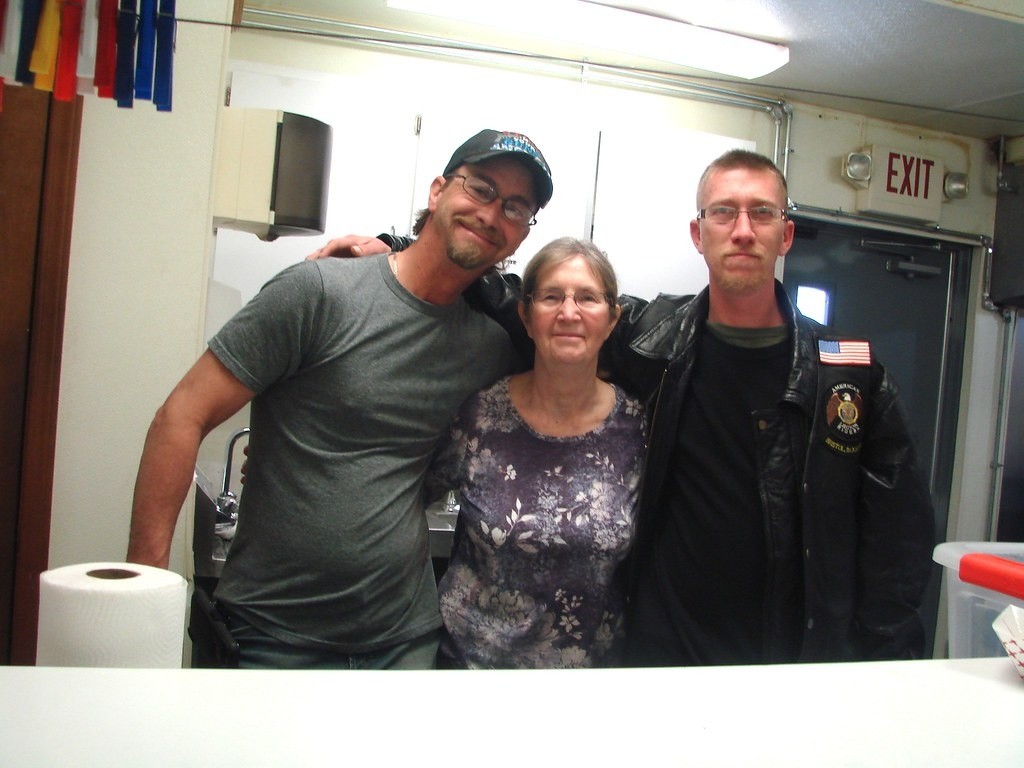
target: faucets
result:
[216,425,270,522]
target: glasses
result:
[698,206,788,224]
[443,174,537,227]
[527,289,615,313]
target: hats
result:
[442,129,554,209]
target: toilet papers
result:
[34,561,189,669]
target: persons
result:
[304,149,937,668]
[239,236,651,669]
[126,127,615,670]
